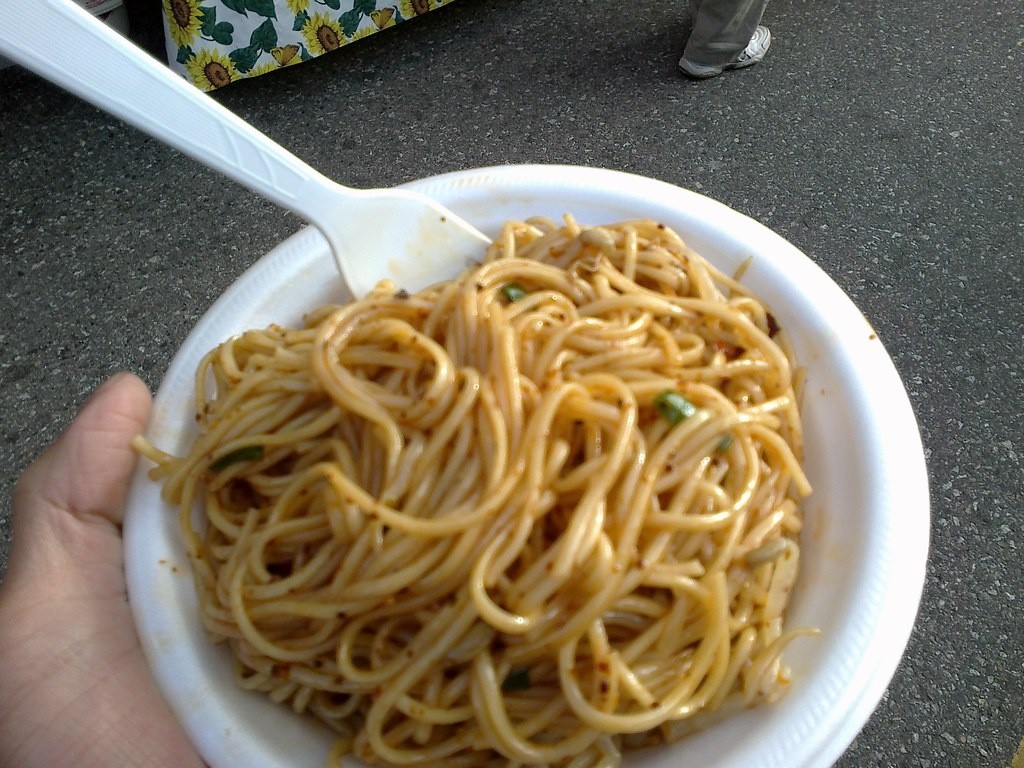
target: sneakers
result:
[679,26,772,78]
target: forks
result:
[0,0,494,303]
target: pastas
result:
[130,213,822,768]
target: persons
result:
[675,0,774,81]
[0,372,221,768]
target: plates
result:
[122,163,931,768]
